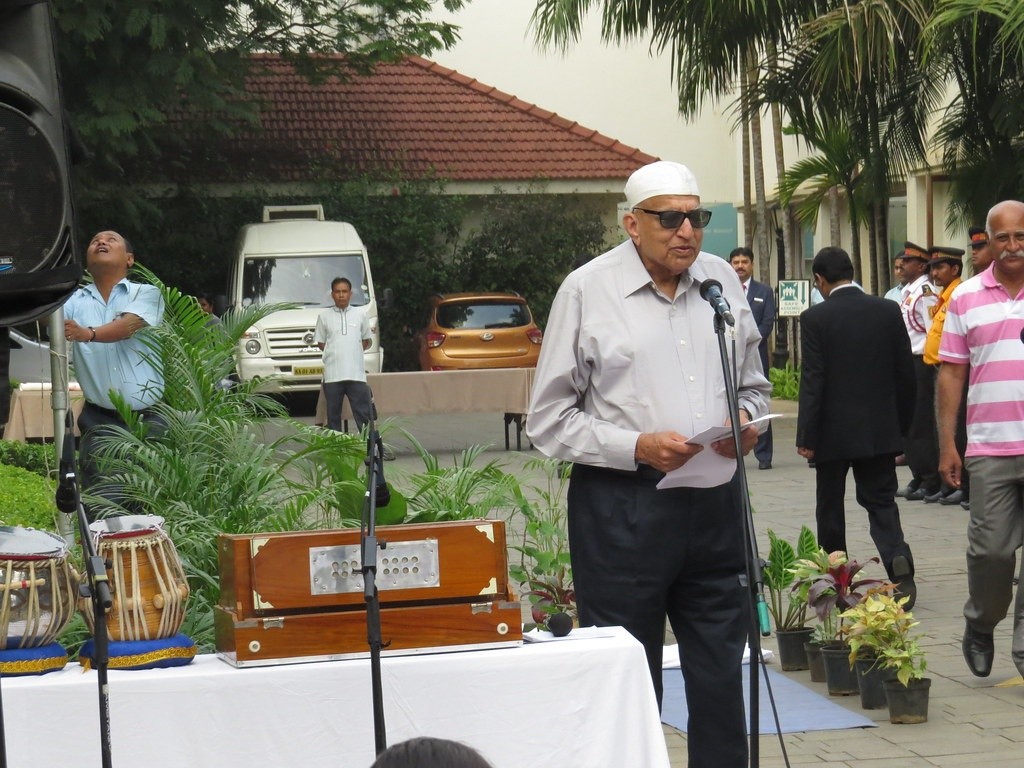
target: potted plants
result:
[760,525,932,725]
[508,451,578,627]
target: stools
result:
[504,412,533,450]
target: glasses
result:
[632,207,713,230]
[812,273,823,289]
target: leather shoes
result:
[923,491,943,503]
[904,486,939,500]
[963,621,995,677]
[894,486,914,497]
[960,500,970,509]
[938,489,968,504]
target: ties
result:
[742,285,746,293]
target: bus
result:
[216,202,386,418]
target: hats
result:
[928,247,966,265]
[900,242,929,261]
[624,160,700,211]
[967,226,988,246]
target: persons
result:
[196,293,223,330]
[524,162,778,768]
[38,230,179,522]
[795,247,919,613]
[884,200,1024,679]
[369,737,493,768]
[315,276,382,439]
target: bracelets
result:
[87,327,95,342]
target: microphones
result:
[367,396,390,508]
[700,279,735,326]
[55,407,81,513]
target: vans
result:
[409,289,544,373]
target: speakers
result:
[0,0,78,329]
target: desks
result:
[3,388,85,443]
[0,625,670,768]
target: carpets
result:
[661,665,877,734]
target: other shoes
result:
[809,462,816,468]
[890,556,917,611]
[759,462,772,469]
[896,454,910,466]
